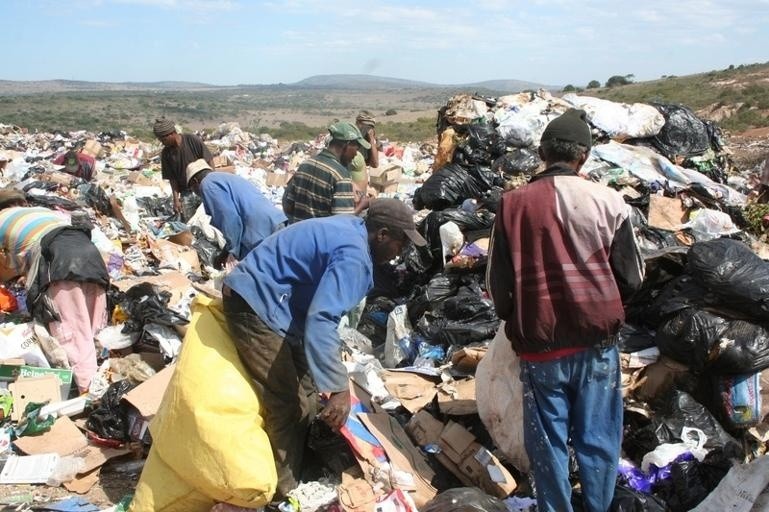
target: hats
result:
[0,190,26,204]
[329,121,372,150]
[367,197,427,247]
[154,116,176,136]
[541,110,590,150]
[186,159,213,187]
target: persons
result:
[486,107,641,512]
[153,117,215,224]
[281,120,374,223]
[220,195,428,512]
[354,111,383,169]
[52,150,95,181]
[324,133,368,202]
[0,189,109,395]
[186,158,289,269]
